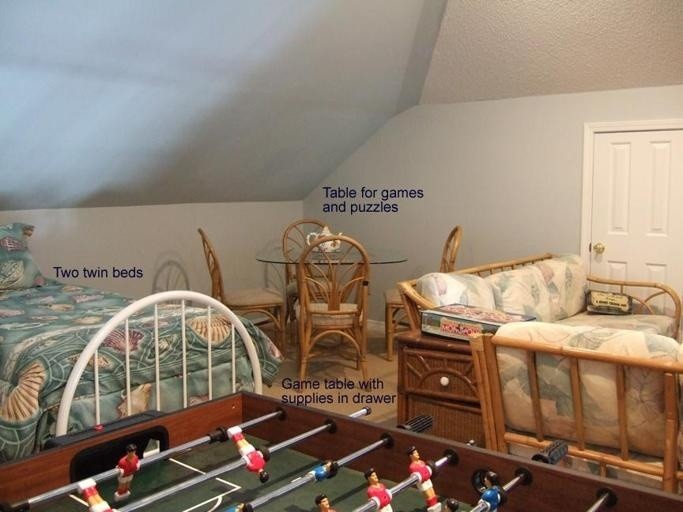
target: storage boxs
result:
[418,301,530,342]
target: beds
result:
[0,275,283,464]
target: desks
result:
[253,244,407,362]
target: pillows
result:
[0,220,45,290]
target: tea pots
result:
[305,225,343,254]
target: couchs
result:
[392,248,682,342]
[464,319,682,497]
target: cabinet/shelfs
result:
[392,328,484,450]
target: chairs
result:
[193,223,287,359]
[288,234,375,393]
[381,223,464,364]
[274,217,351,350]
